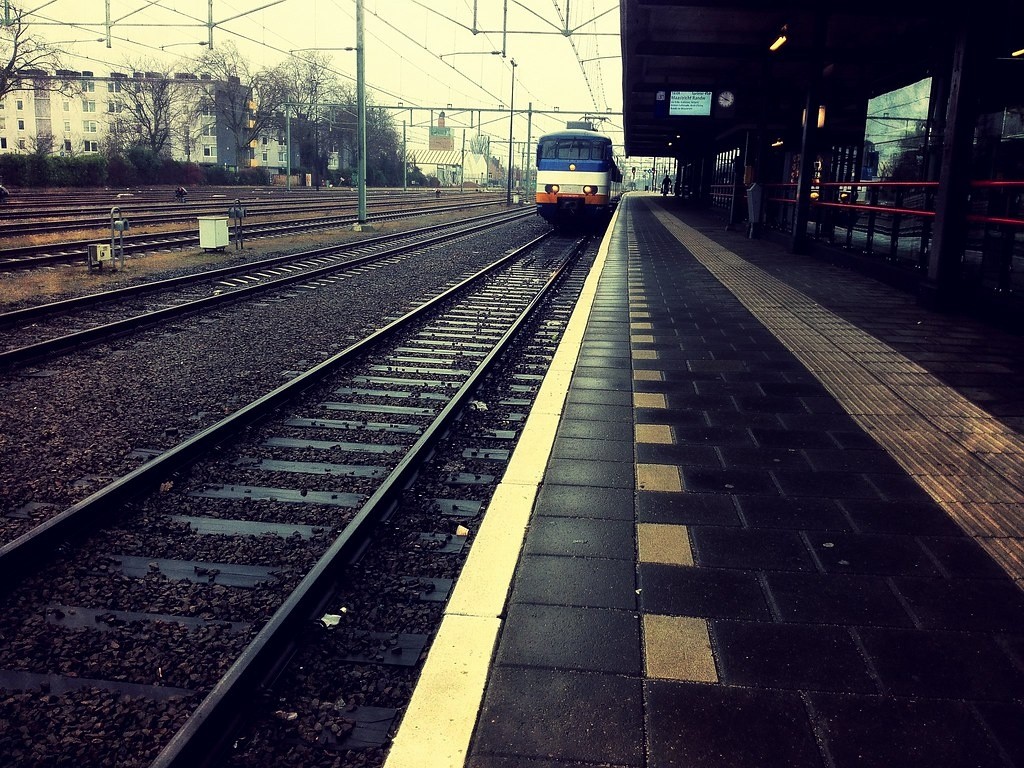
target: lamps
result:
[769,24,789,52]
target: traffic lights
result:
[632,167,635,173]
[648,170,651,173]
[652,168,655,173]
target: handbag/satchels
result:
[661,188,663,194]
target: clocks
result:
[718,91,735,108]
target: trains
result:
[807,139,881,224]
[535,129,624,228]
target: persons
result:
[662,175,672,196]
